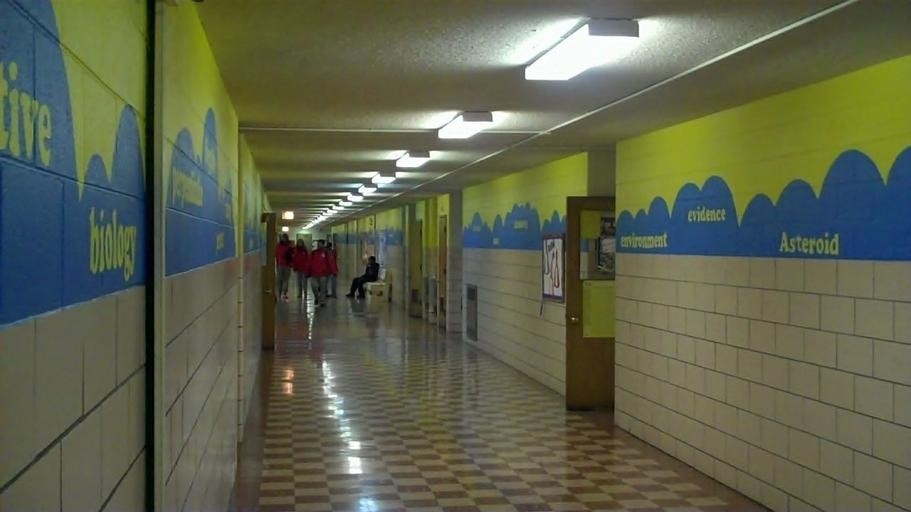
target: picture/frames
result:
[542,234,566,304]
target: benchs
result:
[361,268,392,303]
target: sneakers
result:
[346,292,366,299]
[280,292,338,307]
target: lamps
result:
[281,210,294,232]
[372,172,397,185]
[396,150,429,167]
[304,200,353,230]
[526,21,638,80]
[358,185,378,193]
[347,194,364,200]
[437,111,492,140]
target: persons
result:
[346,256,381,300]
[326,242,341,299]
[309,239,328,308]
[318,238,330,298]
[275,232,294,300]
[288,237,311,301]
